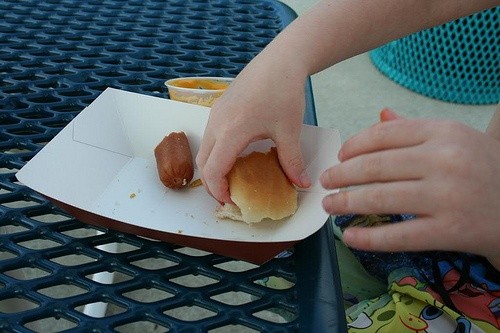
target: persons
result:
[195,0,499,270]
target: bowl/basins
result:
[164,77,238,105]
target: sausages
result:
[152,131,194,190]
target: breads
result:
[216,147,298,222]
